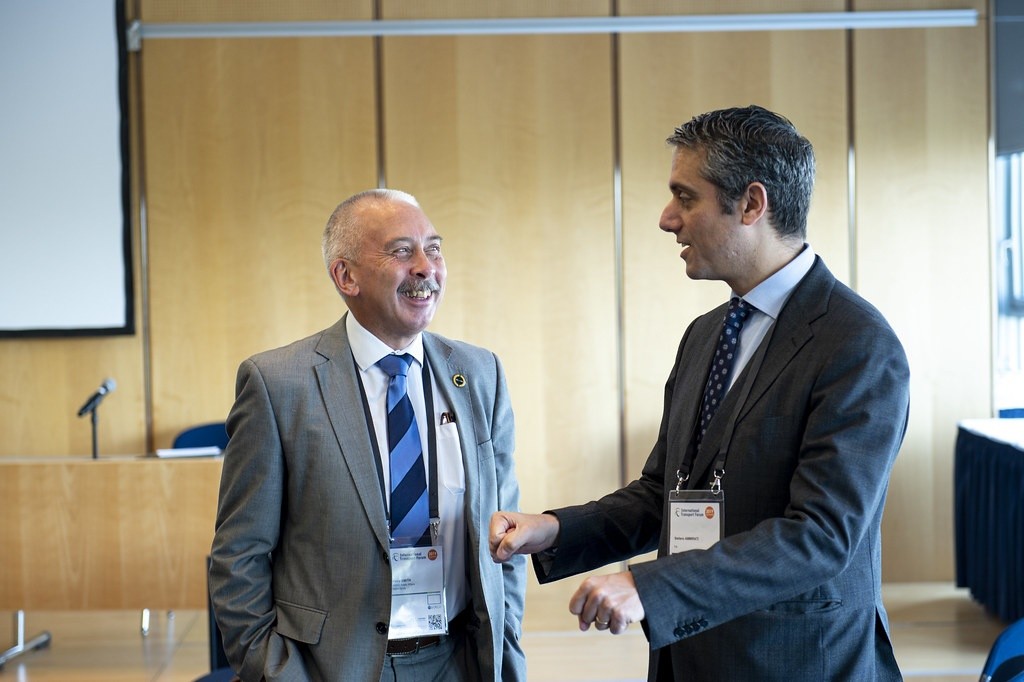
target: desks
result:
[953,418,1024,629]
[0,458,223,670]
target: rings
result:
[596,617,608,626]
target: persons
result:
[488,106,912,681]
[207,189,527,682]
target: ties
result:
[375,353,432,550]
[697,298,759,459]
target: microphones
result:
[78,376,117,419]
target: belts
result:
[386,627,448,655]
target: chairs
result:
[142,422,229,638]
